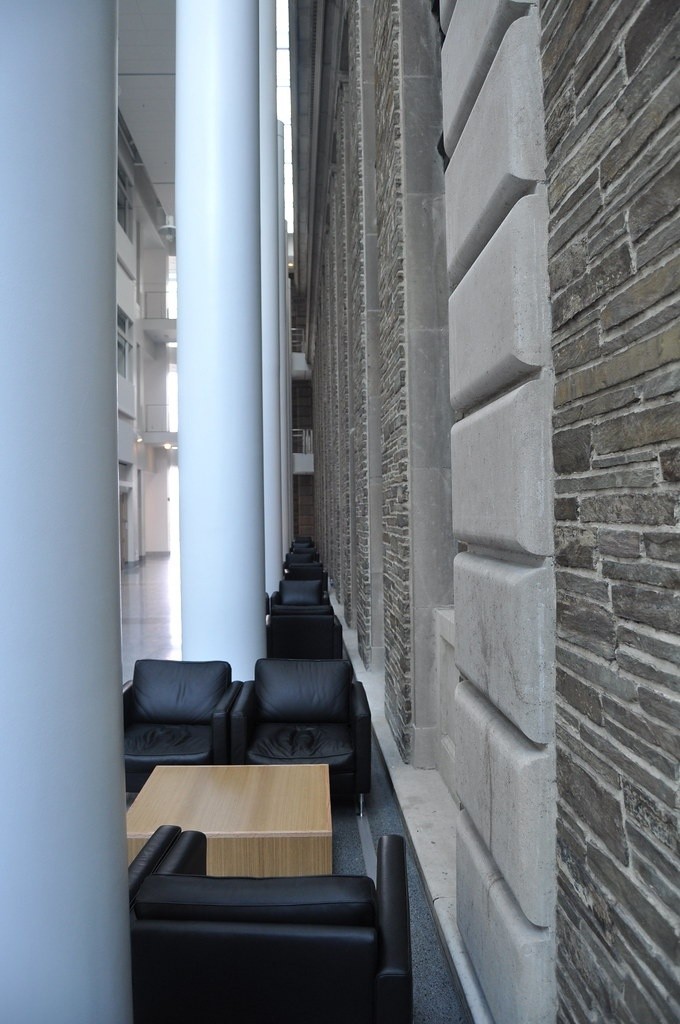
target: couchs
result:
[120,659,242,791]
[232,658,372,817]
[264,536,342,659]
[129,824,416,1024]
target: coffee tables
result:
[127,765,333,877]
